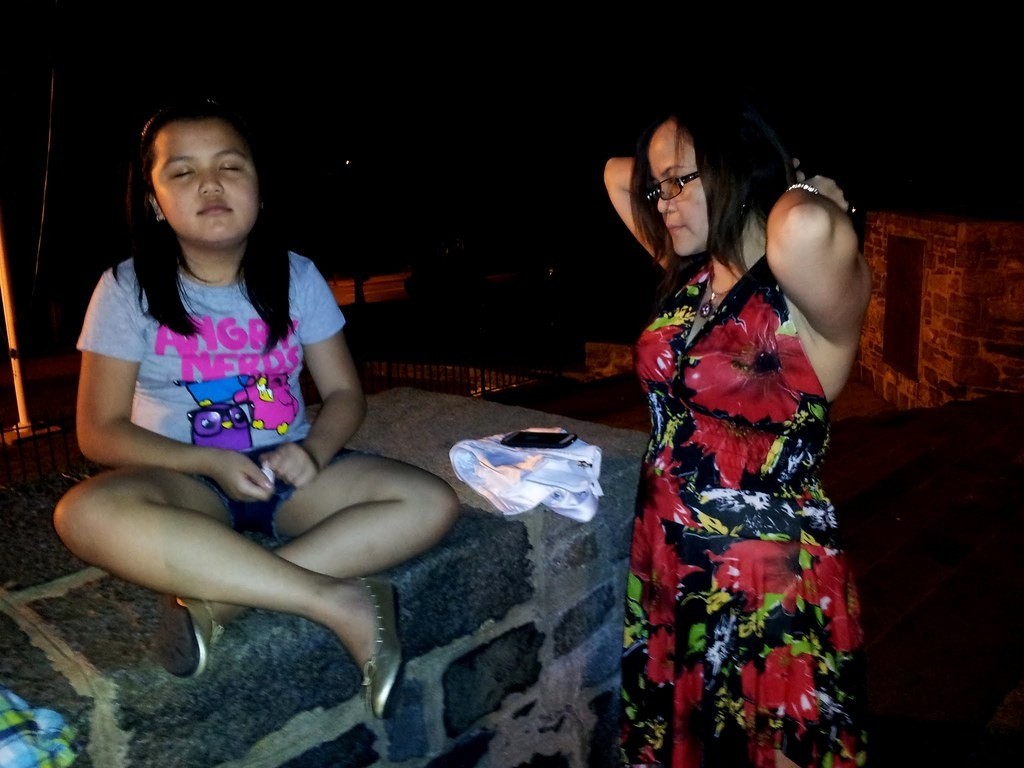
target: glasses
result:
[646,171,700,202]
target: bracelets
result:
[786,183,819,194]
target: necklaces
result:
[699,272,740,318]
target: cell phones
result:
[501,430,577,449]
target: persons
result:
[604,99,872,768]
[54,102,459,720]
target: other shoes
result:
[358,580,405,719]
[156,595,222,679]
